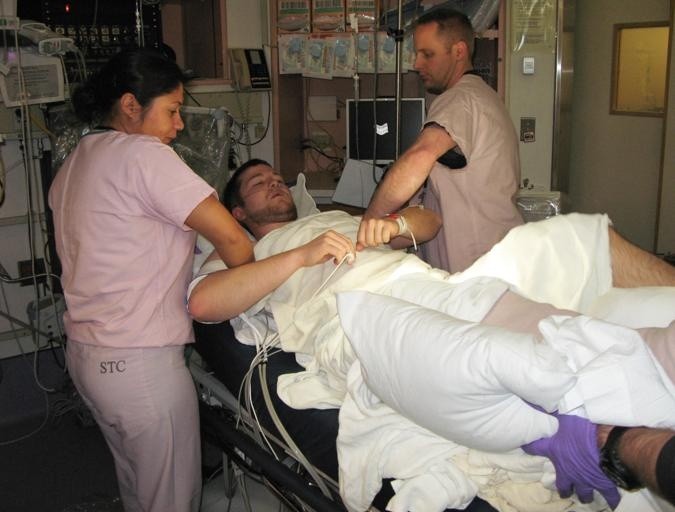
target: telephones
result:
[228,47,271,89]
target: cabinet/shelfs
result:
[268,1,566,216]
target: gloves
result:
[521,400,621,511]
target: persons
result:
[362,8,527,273]
[47,42,257,511]
[188,158,675,503]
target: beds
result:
[187,324,675,512]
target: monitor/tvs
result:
[346,98,425,165]
